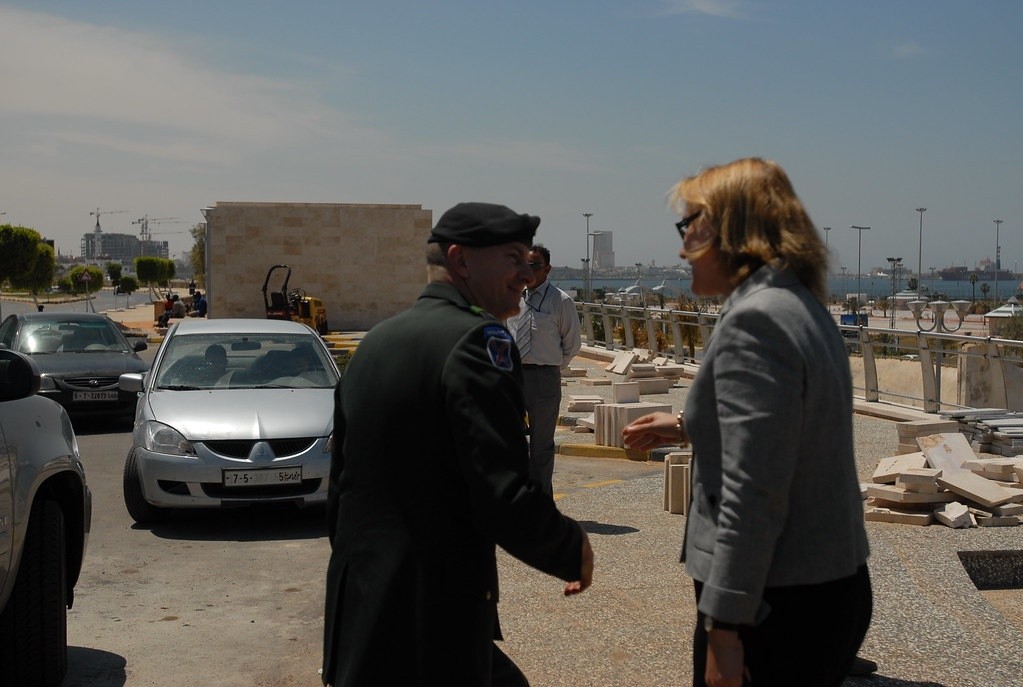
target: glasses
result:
[675,211,703,241]
[528,261,547,272]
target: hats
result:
[427,202,540,249]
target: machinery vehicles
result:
[262,265,328,335]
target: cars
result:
[123,319,344,523]
[0,348,92,676]
[0,312,151,416]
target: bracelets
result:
[676,409,687,449]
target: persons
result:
[323,203,593,687]
[290,347,308,372]
[202,344,228,385]
[621,159,873,687]
[154,279,207,326]
[502,245,580,502]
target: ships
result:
[938,257,1015,281]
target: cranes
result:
[138,218,178,221]
[132,222,189,224]
[140,232,183,235]
[90,211,130,216]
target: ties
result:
[516,291,536,359]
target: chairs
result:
[58,333,73,351]
[263,350,293,375]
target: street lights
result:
[993,219,1003,306]
[582,214,593,291]
[587,232,603,293]
[824,228,831,248]
[849,225,871,325]
[915,208,927,300]
